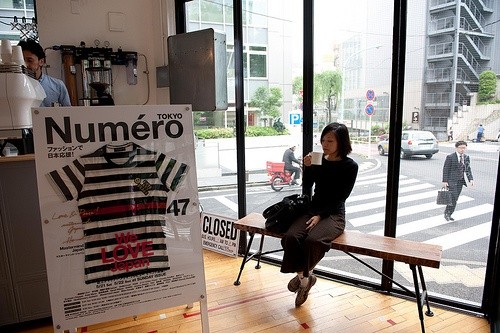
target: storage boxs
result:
[266,160,285,173]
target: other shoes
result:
[288,269,317,306]
[444,214,454,221]
[293,179,300,185]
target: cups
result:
[1,40,24,64]
[307,152,323,165]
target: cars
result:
[378,130,440,159]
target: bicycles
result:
[265,161,304,192]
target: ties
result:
[460,155,463,163]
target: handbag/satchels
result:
[262,194,308,232]
[437,186,452,204]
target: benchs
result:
[234,213,443,333]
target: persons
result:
[280,122,358,308]
[282,146,302,186]
[447,127,453,141]
[476,124,485,142]
[18,38,72,107]
[497,129,500,139]
[442,141,474,222]
